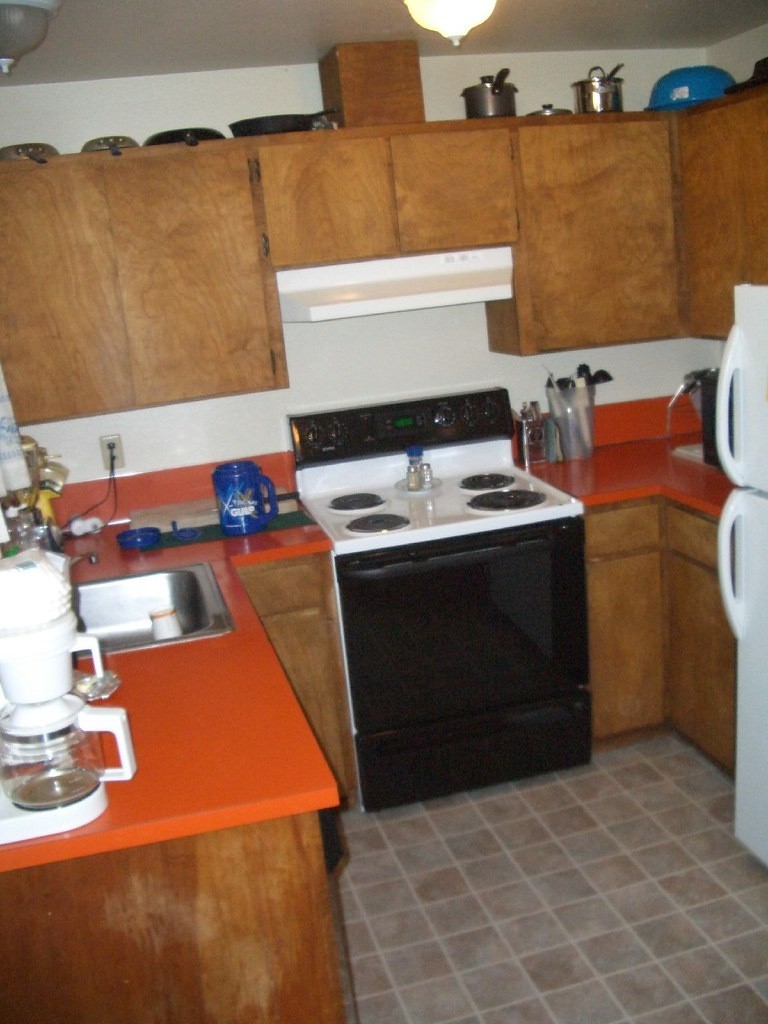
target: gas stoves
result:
[296,439,586,555]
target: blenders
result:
[0,548,137,848]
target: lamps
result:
[0,0,63,73]
[402,0,497,47]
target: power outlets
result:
[99,435,124,470]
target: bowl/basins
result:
[643,65,738,111]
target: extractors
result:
[275,246,513,325]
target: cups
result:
[211,460,280,537]
[546,384,595,461]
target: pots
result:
[228,108,342,138]
[81,136,141,156]
[460,68,519,120]
[571,63,625,113]
[142,127,226,147]
[0,143,61,165]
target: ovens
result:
[334,515,593,812]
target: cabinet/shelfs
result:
[486,110,685,358]
[236,551,360,815]
[663,495,737,783]
[678,86,767,340]
[584,495,669,751]
[252,118,519,273]
[1,808,348,1024]
[0,136,289,425]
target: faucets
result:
[70,551,98,568]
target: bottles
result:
[406,456,433,491]
[148,607,184,641]
[518,402,564,464]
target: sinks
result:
[74,561,236,659]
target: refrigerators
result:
[714,283,768,872]
